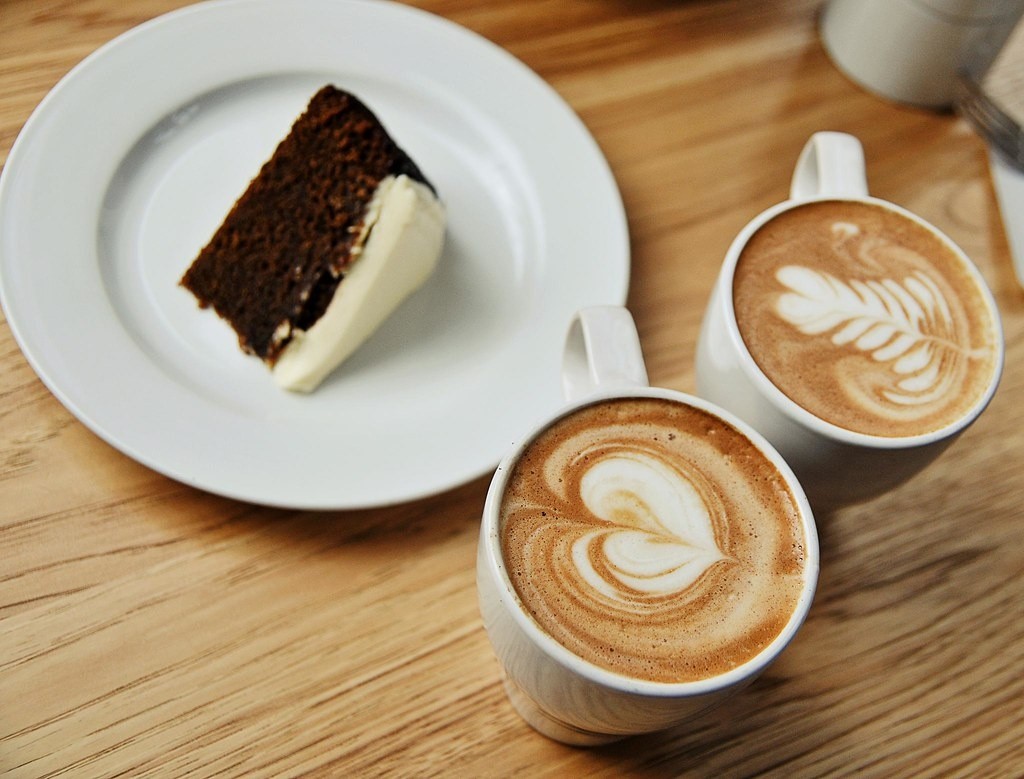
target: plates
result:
[0,0,631,512]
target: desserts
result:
[179,83,448,393]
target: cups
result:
[815,0,1024,108]
[474,305,821,747]
[693,131,1005,514]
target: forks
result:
[948,66,1024,175]
